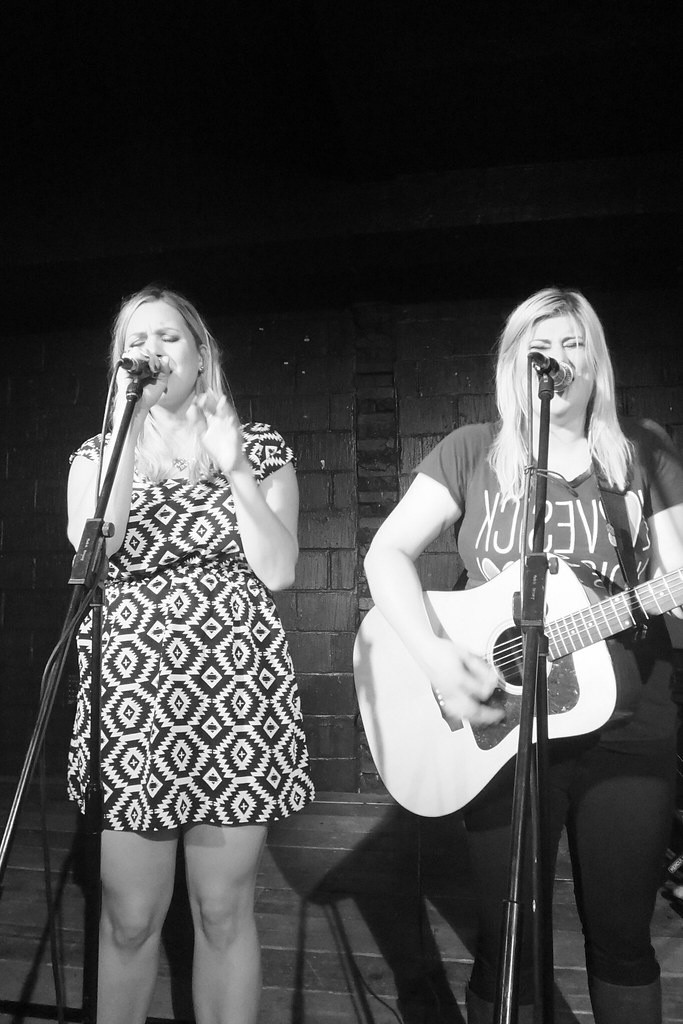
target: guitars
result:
[352,550,683,817]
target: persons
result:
[362,288,683,1023]
[61,289,316,1024]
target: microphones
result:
[527,352,574,392]
[119,358,150,376]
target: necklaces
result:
[172,454,192,472]
